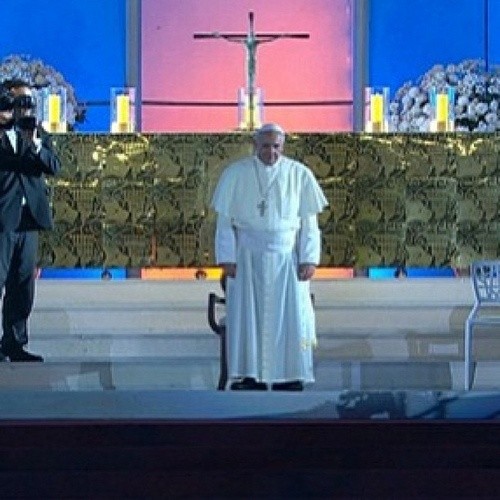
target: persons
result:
[0,79,61,362]
[210,123,329,392]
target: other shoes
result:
[0,349,44,362]
[231,378,267,390]
[272,381,303,390]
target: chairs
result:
[208,273,315,391]
[465,259,500,391]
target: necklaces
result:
[254,159,278,217]
